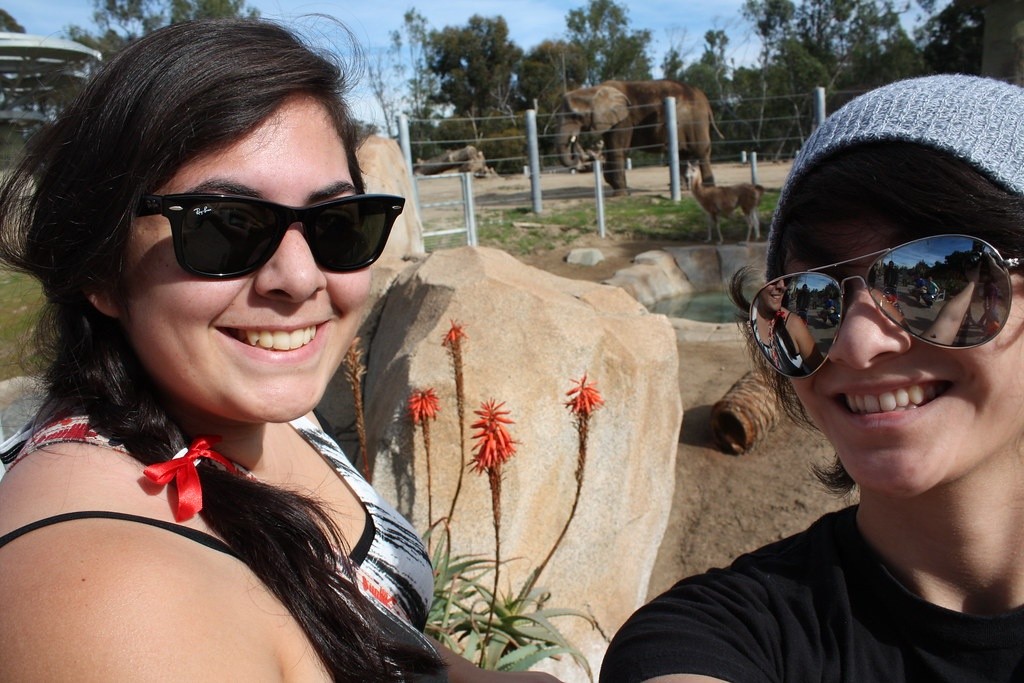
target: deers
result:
[684,158,764,247]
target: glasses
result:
[134,193,405,278]
[770,276,792,287]
[748,238,1023,379]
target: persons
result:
[0,15,561,683]
[750,243,1011,376]
[599,75,1024,683]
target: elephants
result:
[556,78,727,200]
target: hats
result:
[762,72,1024,283]
